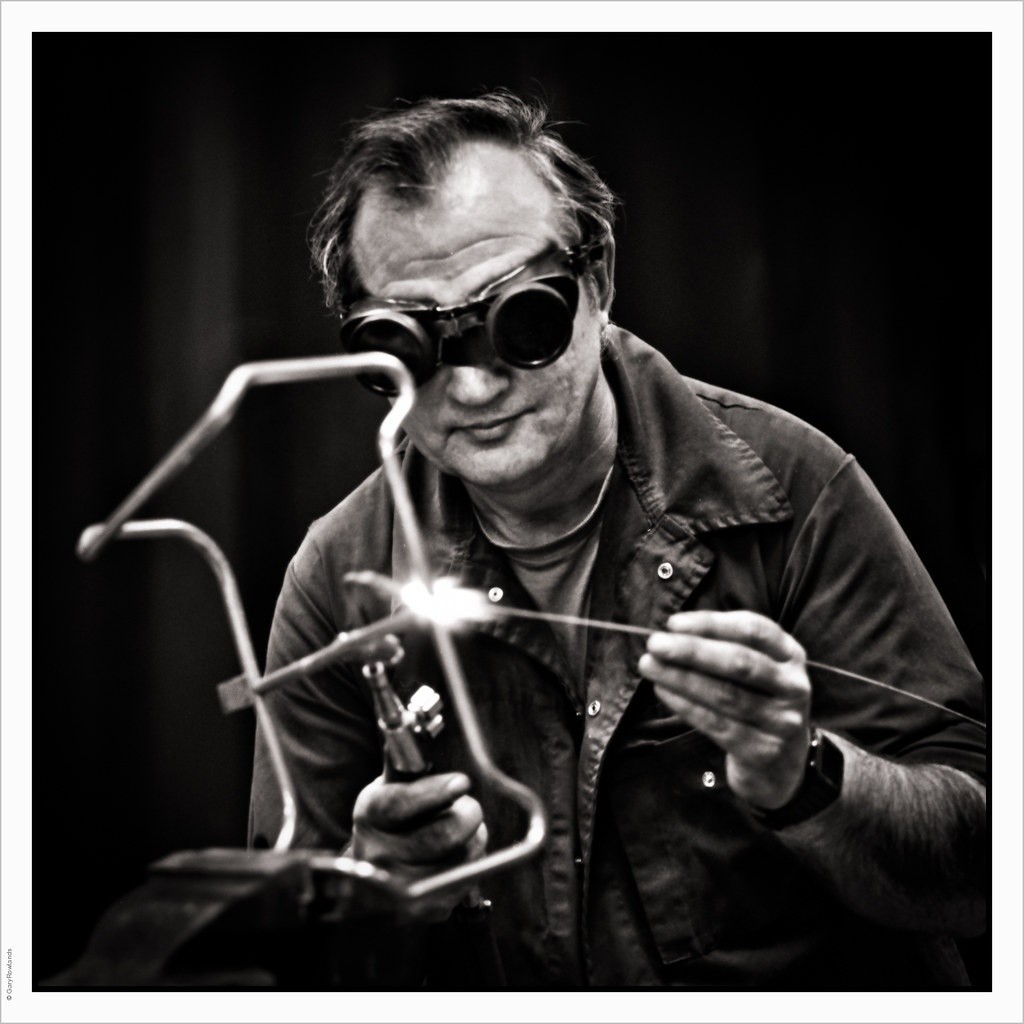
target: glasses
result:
[336,210,602,396]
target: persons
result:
[246,98,987,992]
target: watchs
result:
[750,726,844,832]
